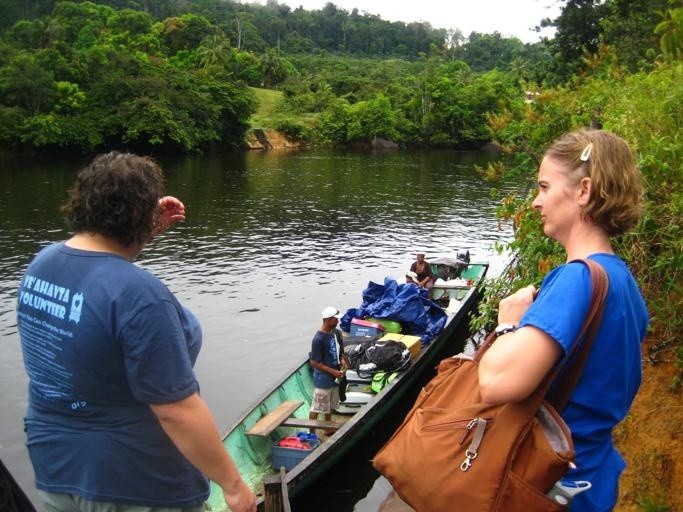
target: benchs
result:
[432,284,472,290]
[283,417,344,429]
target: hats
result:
[407,271,418,282]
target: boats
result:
[206,249,489,512]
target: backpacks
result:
[344,336,411,379]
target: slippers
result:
[324,430,335,436]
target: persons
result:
[478,129,649,512]
[309,307,349,436]
[15,150,257,512]
[406,252,433,289]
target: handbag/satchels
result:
[371,353,575,511]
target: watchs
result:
[495,322,515,338]
